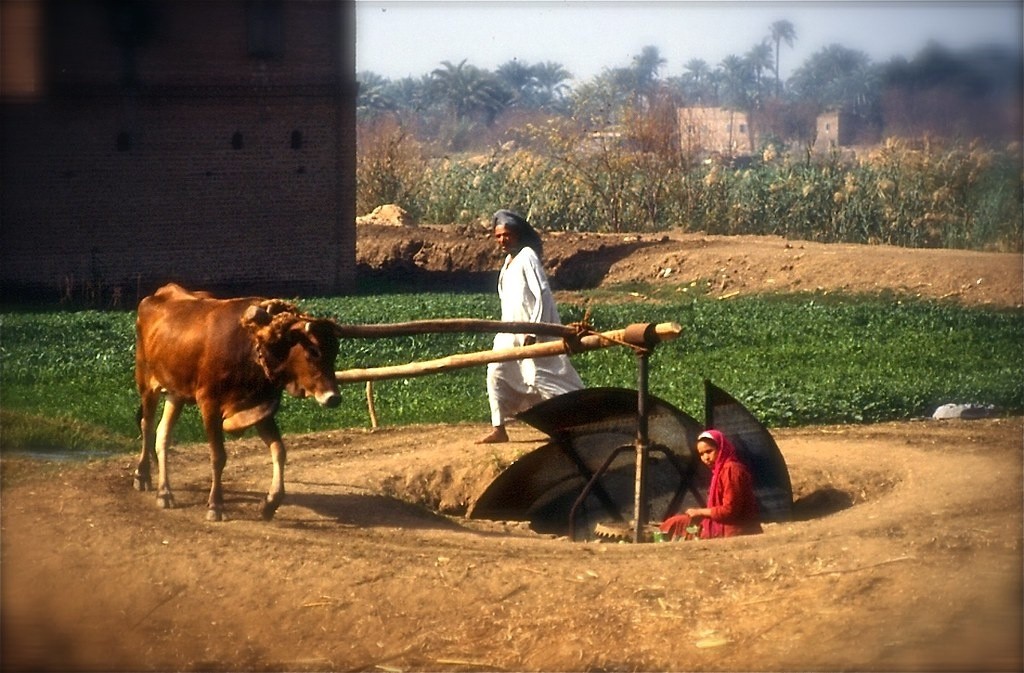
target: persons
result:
[473,209,586,444]
[659,430,763,541]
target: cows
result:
[132,284,347,521]
